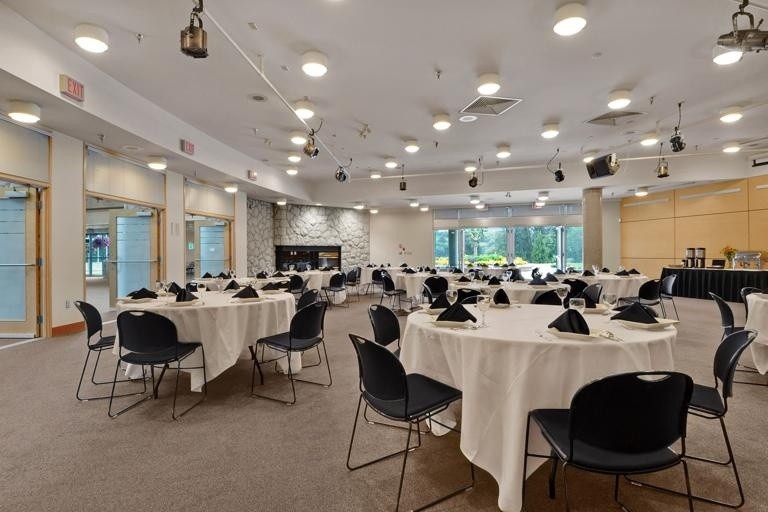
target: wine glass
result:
[307,265,311,272]
[197,282,206,304]
[246,281,256,289]
[566,266,571,277]
[156,282,164,302]
[503,274,508,289]
[253,269,258,280]
[479,271,484,283]
[162,280,172,298]
[422,265,426,274]
[417,267,420,275]
[569,298,586,318]
[594,268,599,278]
[447,291,458,306]
[230,271,236,280]
[507,271,512,285]
[603,293,618,316]
[469,272,475,286]
[437,267,440,275]
[290,265,295,275]
[215,277,223,293]
[557,286,568,313]
[476,294,491,329]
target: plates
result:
[511,280,527,284]
[234,298,263,302]
[485,285,504,288]
[546,282,560,286]
[423,308,445,314]
[584,304,607,316]
[488,303,519,308]
[528,285,550,289]
[258,288,289,294]
[616,276,634,279]
[119,298,153,303]
[454,281,471,285]
[427,321,475,329]
[164,301,202,307]
[547,328,603,341]
[606,319,679,329]
[630,274,643,277]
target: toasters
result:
[592,264,596,270]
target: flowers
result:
[719,247,739,262]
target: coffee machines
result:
[695,248,706,268]
[682,248,694,267]
